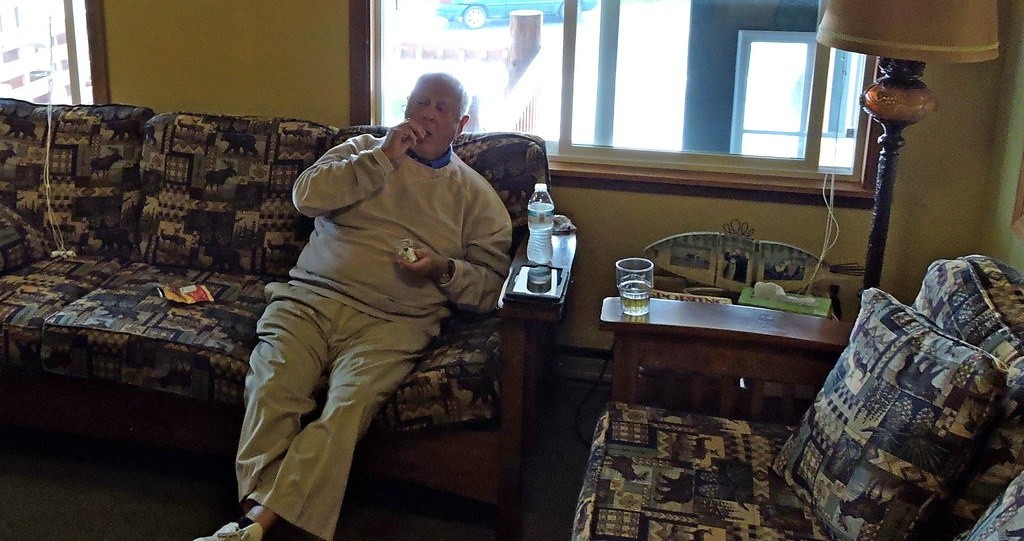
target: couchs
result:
[571,255,1024,541]
[0,97,578,541]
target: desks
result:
[598,296,854,426]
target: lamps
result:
[815,0,998,319]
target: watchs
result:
[439,260,454,285]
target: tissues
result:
[737,281,833,320]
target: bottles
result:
[527,266,552,295]
[527,183,554,264]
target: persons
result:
[194,71,512,541]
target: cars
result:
[435,0,597,27]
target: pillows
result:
[911,257,1024,541]
[771,288,1008,541]
[0,200,51,274]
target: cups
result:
[615,258,654,316]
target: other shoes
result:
[193,522,264,541]
